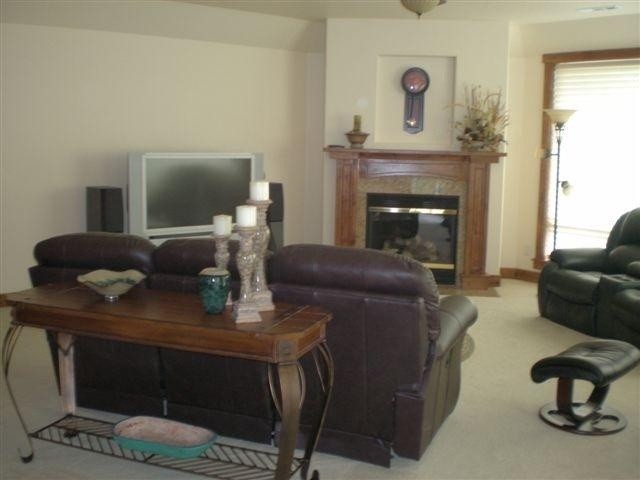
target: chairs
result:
[537,206,640,351]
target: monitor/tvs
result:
[123,151,263,248]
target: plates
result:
[75,269,147,298]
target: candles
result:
[236,206,257,227]
[213,215,232,235]
[249,181,269,200]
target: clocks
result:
[401,67,430,135]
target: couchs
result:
[31,232,479,468]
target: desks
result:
[1,281,334,480]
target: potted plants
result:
[445,82,510,154]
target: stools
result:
[601,275,640,347]
[530,340,640,436]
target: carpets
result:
[461,334,475,363]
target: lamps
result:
[398,0,446,20]
[531,108,576,250]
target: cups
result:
[197,267,234,316]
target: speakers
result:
[251,181,283,222]
[86,186,123,233]
